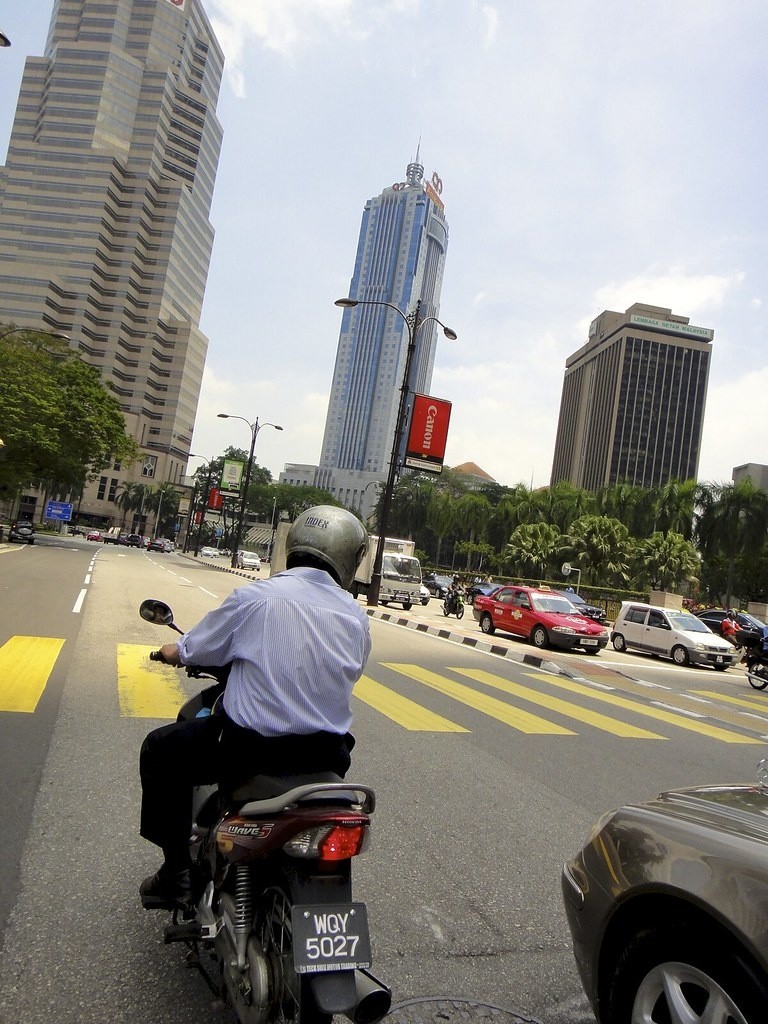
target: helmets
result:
[285,505,369,590]
[454,575,460,581]
[727,609,736,619]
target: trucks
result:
[269,521,424,610]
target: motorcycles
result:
[134,596,395,1024]
[438,584,469,619]
[713,623,768,690]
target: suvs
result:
[127,533,145,549]
[8,519,37,545]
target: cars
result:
[691,609,767,652]
[610,599,740,673]
[422,574,454,599]
[143,536,151,547]
[147,538,165,553]
[465,582,506,605]
[86,531,103,543]
[201,546,233,558]
[118,531,128,546]
[419,583,431,606]
[236,551,261,573]
[68,526,85,535]
[162,539,178,553]
[552,589,607,625]
[104,533,118,545]
[473,585,610,658]
[560,776,767,1024]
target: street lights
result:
[152,489,166,539]
[187,452,215,558]
[179,474,198,553]
[333,293,460,610]
[217,413,284,570]
[265,497,277,564]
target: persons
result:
[722,609,743,646]
[401,562,413,575]
[139,505,372,909]
[83,529,88,539]
[566,585,574,593]
[453,571,459,577]
[763,625,768,659]
[483,573,493,583]
[425,570,437,575]
[475,576,481,583]
[445,574,465,607]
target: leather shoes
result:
[139,866,191,901]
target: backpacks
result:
[719,621,729,636]
